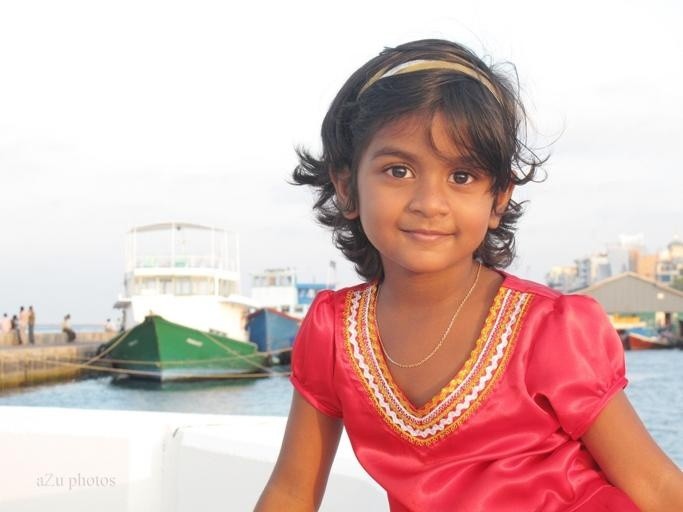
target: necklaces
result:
[372,256,482,369]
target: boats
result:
[106,223,336,382]
[621,328,674,349]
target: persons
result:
[248,36,683,512]
[102,318,113,335]
[18,306,29,345]
[0,312,11,335]
[59,314,75,345]
[9,314,23,345]
[27,305,36,345]
